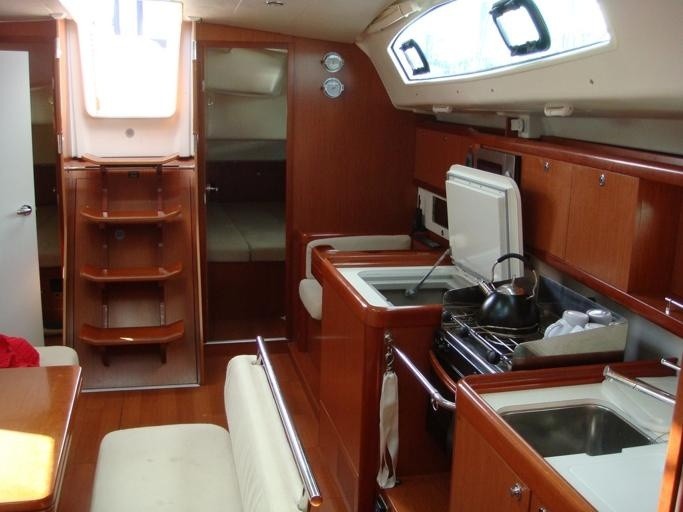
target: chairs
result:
[90,334,321,512]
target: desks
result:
[1,365,86,510]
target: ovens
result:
[426,335,490,446]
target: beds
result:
[205,198,287,319]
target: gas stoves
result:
[444,298,550,369]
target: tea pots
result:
[480,250,539,334]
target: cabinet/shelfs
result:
[413,128,480,195]
[517,152,682,296]
[449,381,595,511]
[76,152,187,367]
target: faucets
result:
[602,363,679,409]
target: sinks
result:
[495,398,658,461]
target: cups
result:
[547,310,609,336]
